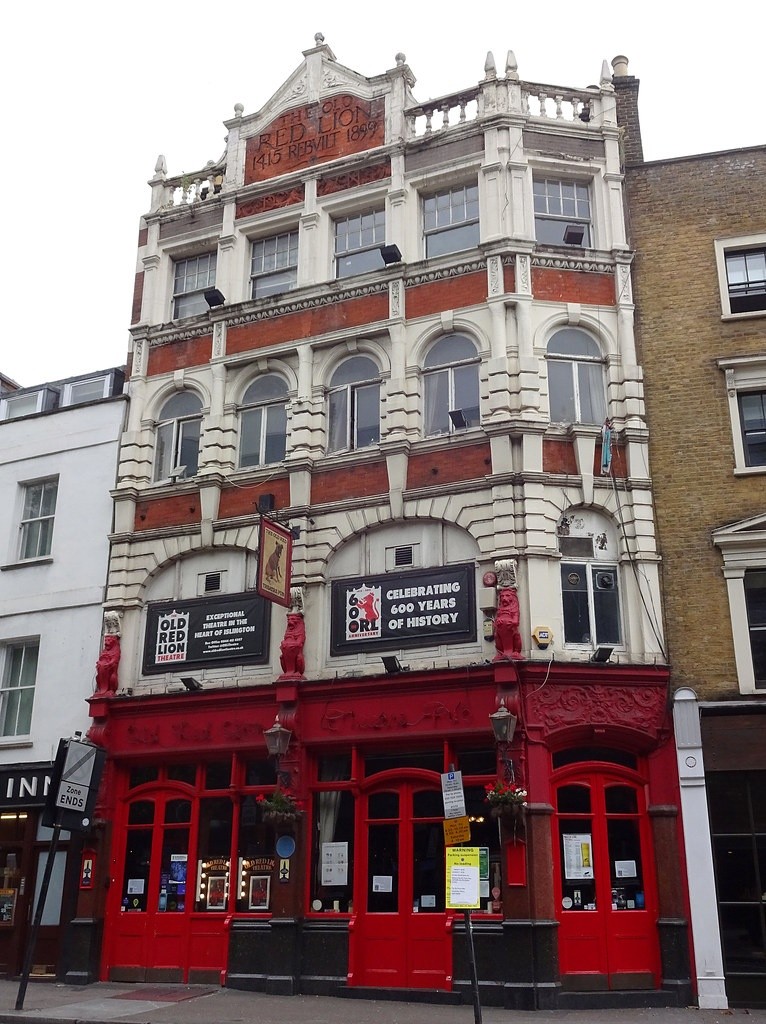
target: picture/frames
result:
[249,876,269,910]
[207,877,226,909]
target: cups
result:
[334,901,339,912]
[487,901,493,915]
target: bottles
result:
[158,886,167,913]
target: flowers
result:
[484,782,526,805]
[255,787,306,821]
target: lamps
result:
[180,678,203,690]
[447,408,470,430]
[204,290,225,309]
[382,655,401,672]
[563,226,584,245]
[592,647,613,662]
[381,245,402,264]
[489,698,517,742]
[264,716,289,755]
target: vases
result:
[492,801,523,818]
[271,821,294,836]
